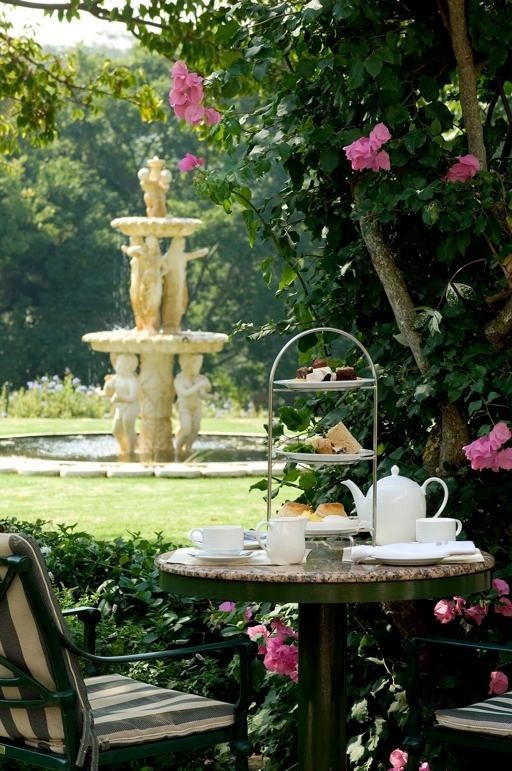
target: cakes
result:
[304,421,361,454]
[296,359,356,381]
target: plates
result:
[187,551,258,565]
[370,552,450,567]
[304,522,361,537]
[273,448,373,465]
[274,378,378,394]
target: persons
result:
[103,354,212,456]
[120,236,209,334]
[137,168,173,217]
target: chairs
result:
[406,633,511,769]
[0,530,258,771]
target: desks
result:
[152,542,496,770]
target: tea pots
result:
[340,465,450,546]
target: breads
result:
[277,502,346,521]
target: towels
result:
[349,541,476,566]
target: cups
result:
[415,517,462,542]
[255,517,305,567]
[188,527,245,554]
